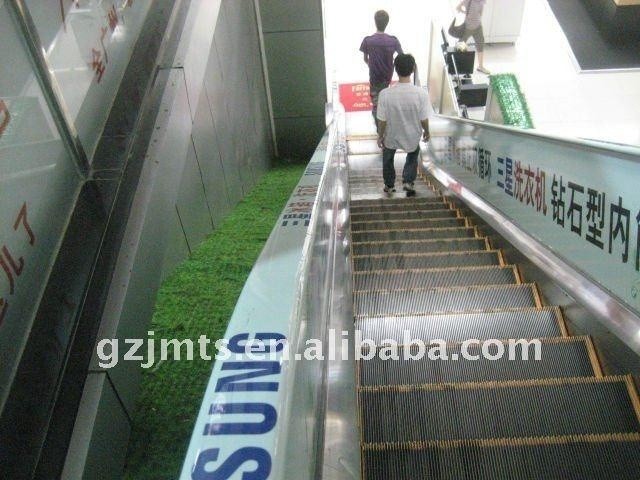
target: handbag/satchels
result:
[448,17,465,37]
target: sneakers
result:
[477,67,490,74]
[402,182,415,192]
[383,185,396,192]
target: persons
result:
[457,0,490,74]
[359,10,404,133]
[377,54,430,193]
